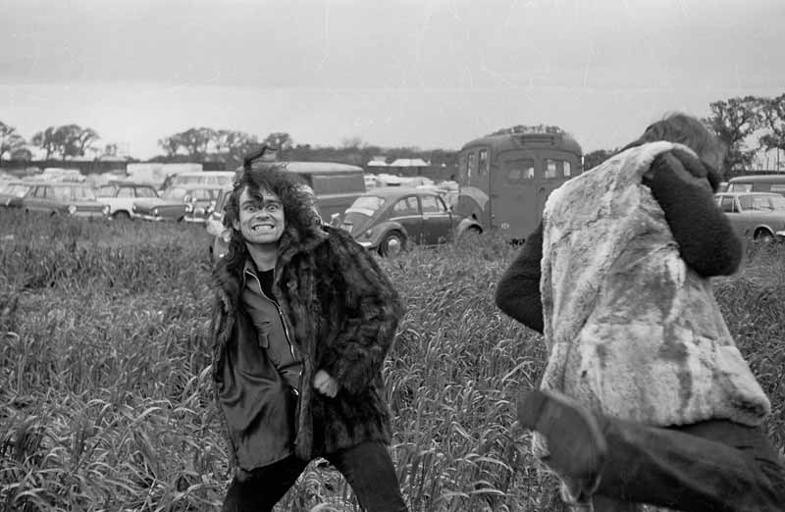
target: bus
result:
[455,120,585,243]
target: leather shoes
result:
[517,387,608,477]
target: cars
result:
[271,161,460,226]
[708,166,785,242]
[0,161,237,224]
[338,187,483,259]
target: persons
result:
[209,145,411,511]
[497,113,784,510]
